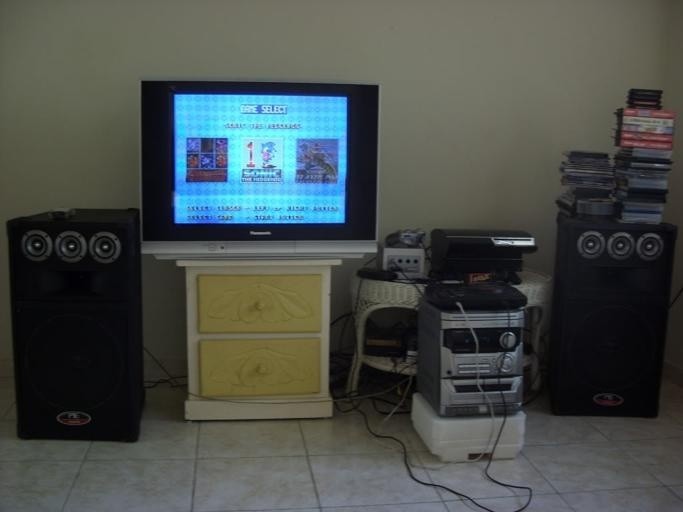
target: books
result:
[554,88,680,227]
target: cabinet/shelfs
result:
[173,260,342,422]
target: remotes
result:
[356,267,397,281]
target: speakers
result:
[545,212,679,419]
[6,208,146,444]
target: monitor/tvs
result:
[137,78,382,258]
